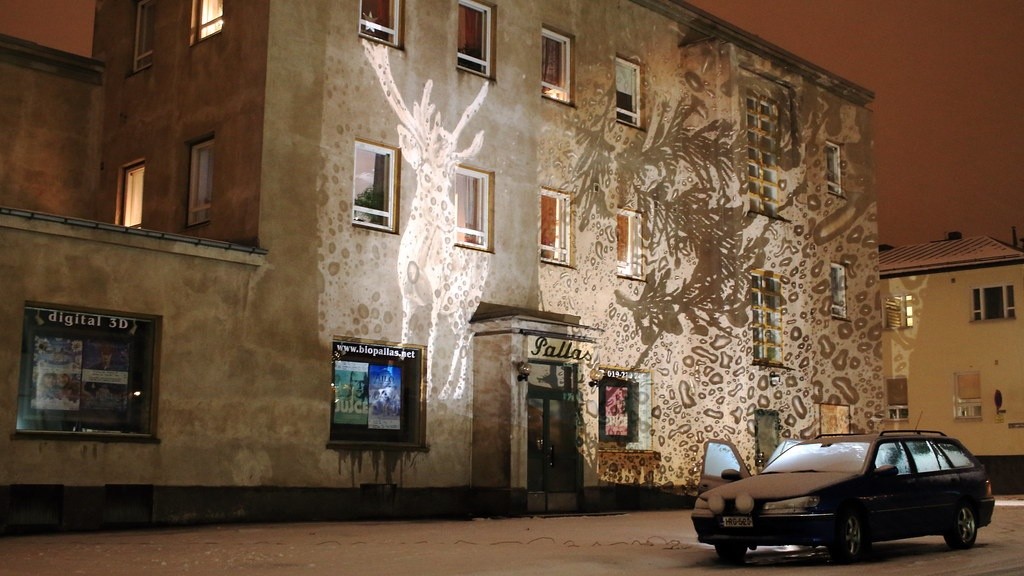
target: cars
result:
[690,408,996,566]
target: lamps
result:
[587,368,604,387]
[768,370,782,388]
[515,362,532,382]
